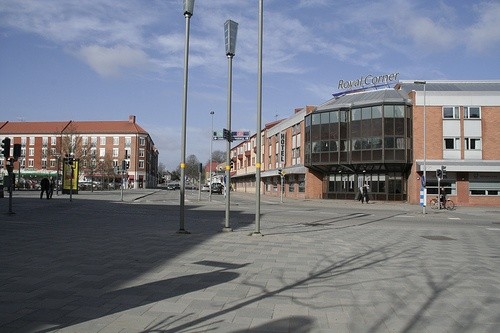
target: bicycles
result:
[430,197,454,210]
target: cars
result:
[167,184,176,190]
[202,186,209,192]
[211,183,224,195]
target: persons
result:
[361,183,368,203]
[437,186,446,209]
[40,176,55,199]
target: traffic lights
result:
[1,137,10,158]
[441,166,447,175]
[436,169,441,177]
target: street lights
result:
[209,111,214,202]
